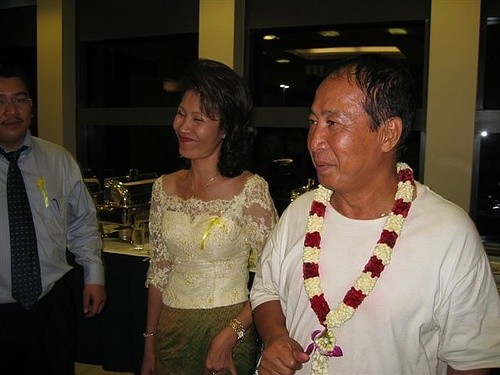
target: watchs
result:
[228,317,245,342]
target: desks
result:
[66,218,151,375]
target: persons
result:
[249,57,500,375]
[144,59,280,375]
[0,68,106,375]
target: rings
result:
[212,371,220,375]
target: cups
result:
[133,223,146,250]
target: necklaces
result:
[303,162,415,375]
[186,169,220,199]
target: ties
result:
[1,145,43,310]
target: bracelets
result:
[143,332,158,338]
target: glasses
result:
[0,98,32,108]
